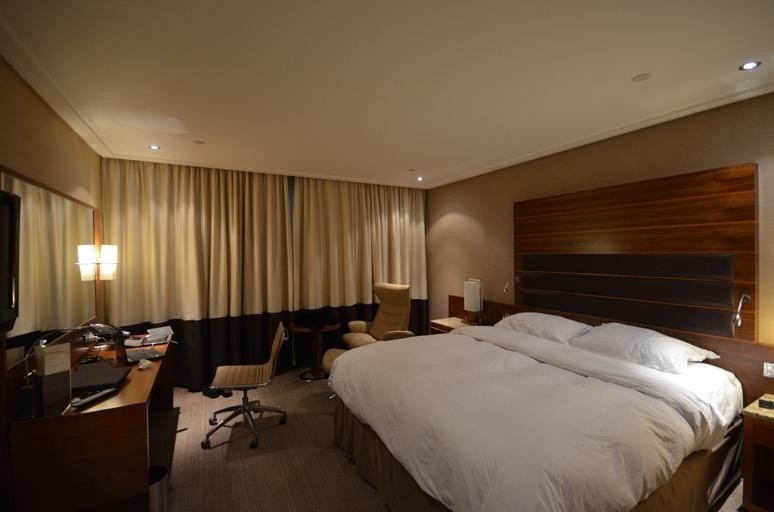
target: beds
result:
[332,304,773,512]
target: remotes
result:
[71,387,118,408]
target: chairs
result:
[322,282,415,376]
[197,321,288,450]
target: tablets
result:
[140,336,168,346]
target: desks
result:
[1,329,181,511]
[290,321,343,382]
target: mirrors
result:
[0,166,105,373]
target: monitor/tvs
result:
[0,188,21,331]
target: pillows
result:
[491,306,720,377]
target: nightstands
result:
[739,390,774,512]
[429,315,479,336]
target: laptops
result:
[110,324,165,366]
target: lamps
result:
[73,244,95,284]
[96,243,119,283]
[460,279,484,325]
[503,276,521,296]
[732,291,754,330]
